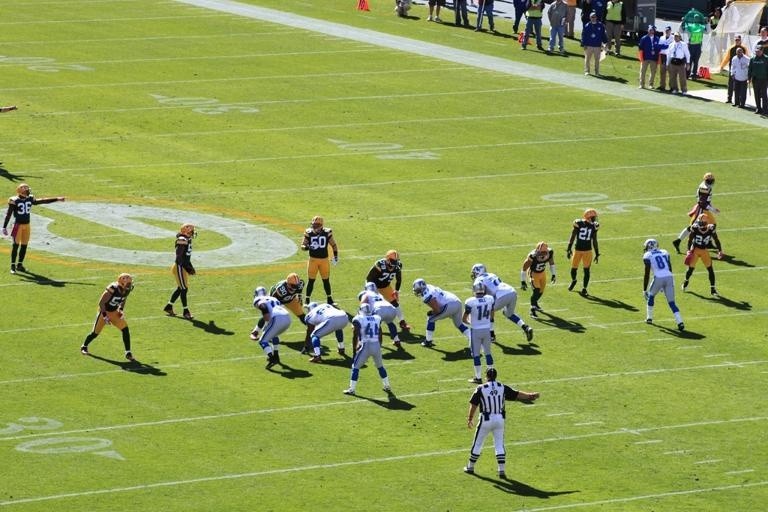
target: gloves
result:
[103,316,112,325]
[118,310,124,320]
[3,228,8,235]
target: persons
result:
[520,241,556,317]
[453,1,470,28]
[0,105,17,113]
[462,281,495,384]
[252,287,290,369]
[640,7,722,94]
[512,1,569,55]
[396,1,411,17]
[413,279,469,347]
[567,209,600,296]
[251,274,308,340]
[427,1,446,23]
[474,1,494,32]
[463,367,540,479]
[578,1,626,77]
[303,302,348,362]
[721,26,768,117]
[301,216,339,305]
[366,250,411,330]
[643,239,685,330]
[163,223,197,320]
[471,264,534,343]
[80,272,135,361]
[344,283,401,394]
[1,183,68,275]
[674,173,723,296]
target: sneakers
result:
[11,264,15,273]
[163,304,176,315]
[16,263,25,272]
[645,239,723,330]
[463,467,474,472]
[251,320,533,396]
[80,346,89,354]
[500,471,504,477]
[125,352,135,361]
[183,309,192,319]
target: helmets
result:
[118,273,134,291]
[180,224,197,239]
[698,214,709,228]
[704,172,715,187]
[16,184,29,198]
